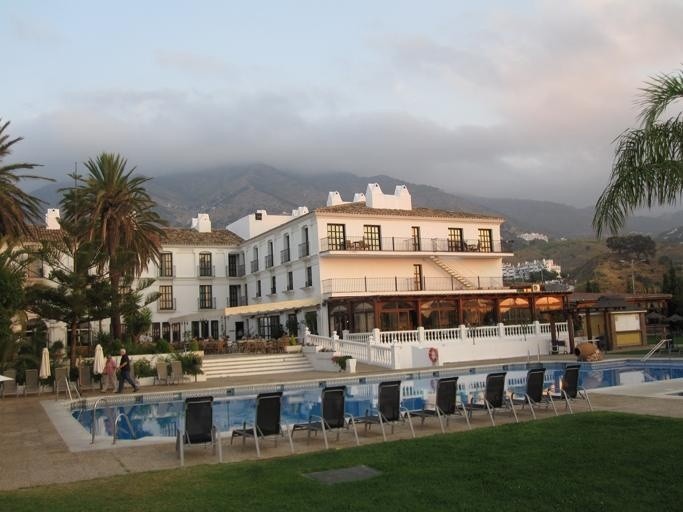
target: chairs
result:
[170,336,290,354]
[506,368,559,421]
[230,391,295,457]
[543,366,593,414]
[404,376,472,434]
[176,396,223,468]
[291,385,360,450]
[353,379,416,441]
[456,372,518,426]
[0,361,190,400]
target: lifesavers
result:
[428,348,438,362]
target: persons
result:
[98,352,117,393]
[114,348,138,393]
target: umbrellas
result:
[92,343,106,375]
[662,313,683,324]
[0,374,14,383]
[645,311,665,327]
[37,346,51,382]
